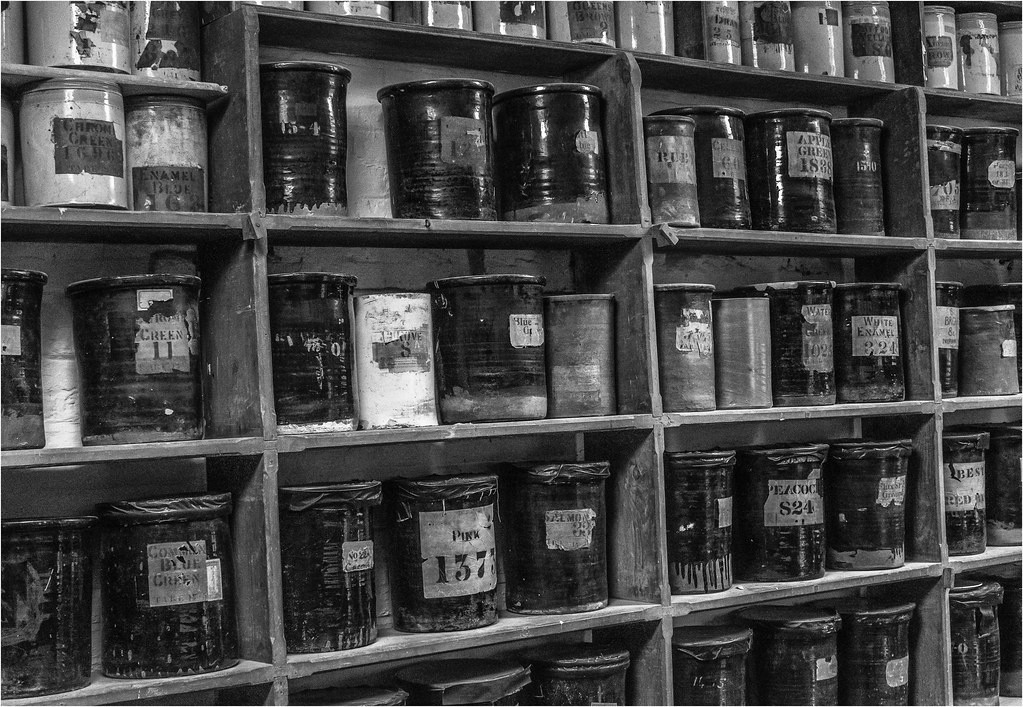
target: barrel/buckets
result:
[1,0,1022,706]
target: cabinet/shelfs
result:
[1,0,1023,707]
